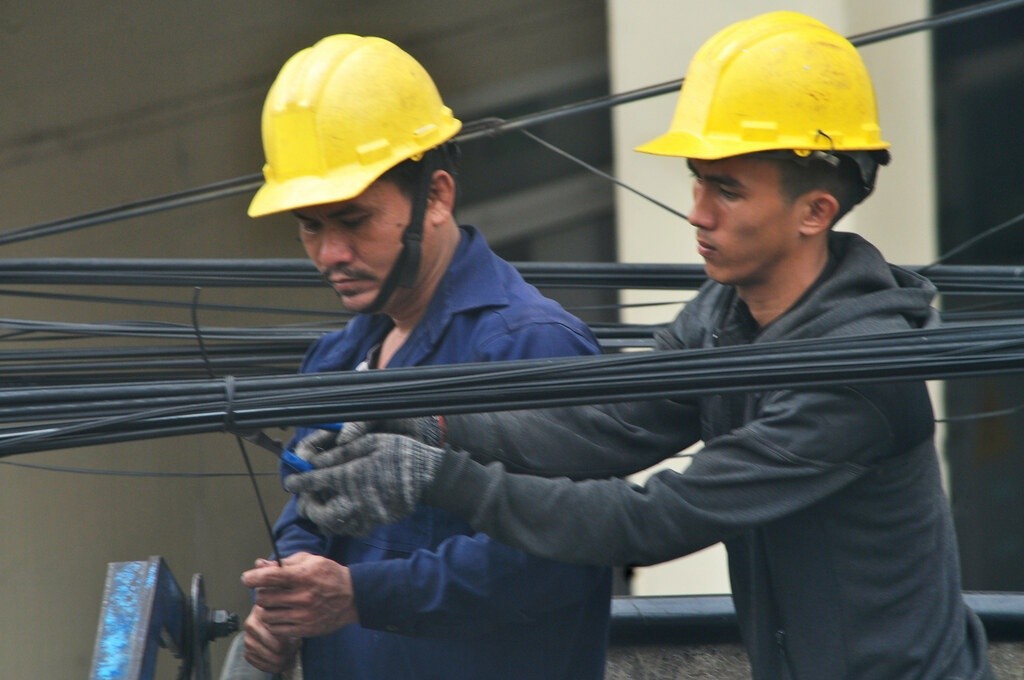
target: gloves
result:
[284,437,445,534]
[285,414,446,462]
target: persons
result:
[240,34,606,680]
[283,9,998,680]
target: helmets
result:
[245,30,464,219]
[630,10,888,163]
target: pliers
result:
[235,420,342,474]
